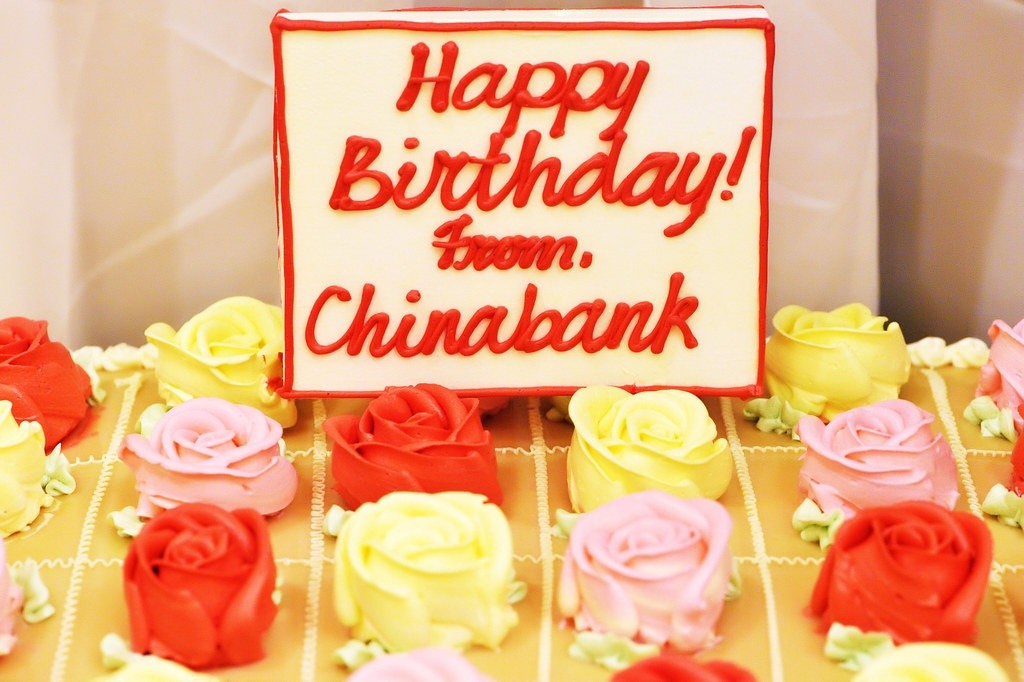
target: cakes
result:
[0,296,1024,682]
